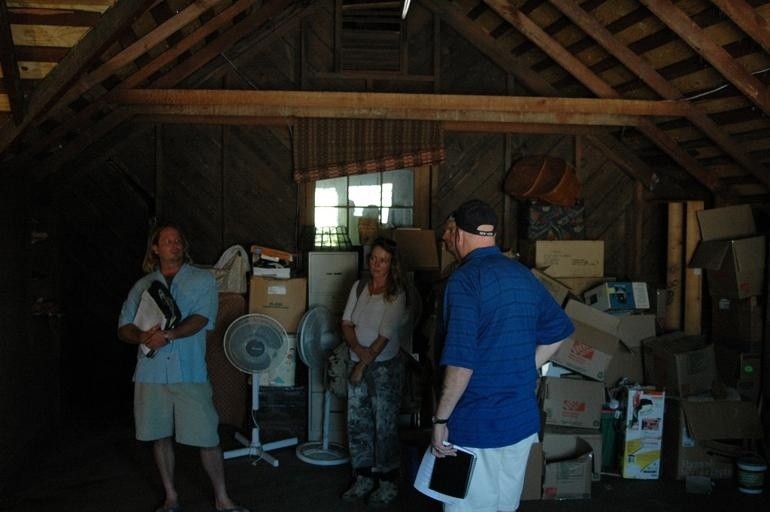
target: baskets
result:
[502,155,582,209]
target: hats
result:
[454,199,499,237]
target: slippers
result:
[217,503,250,512]
[155,503,184,512]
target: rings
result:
[435,447,439,451]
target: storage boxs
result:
[250,243,308,336]
[543,451,591,500]
[685,205,767,301]
[543,423,602,473]
[727,378,761,403]
[528,238,604,277]
[726,348,763,377]
[551,276,615,303]
[670,394,764,482]
[642,333,725,397]
[549,299,656,381]
[538,377,604,429]
[709,296,762,343]
[530,264,571,309]
[520,441,543,500]
[618,382,666,481]
[584,281,651,316]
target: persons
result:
[430,210,462,415]
[414,198,575,512]
[116,220,250,512]
[340,236,407,503]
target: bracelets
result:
[159,329,171,344]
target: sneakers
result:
[367,478,400,512]
[341,474,374,503]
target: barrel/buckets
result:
[737,460,766,495]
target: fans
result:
[222,306,348,467]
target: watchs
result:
[432,415,448,424]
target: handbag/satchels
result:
[327,339,353,399]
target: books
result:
[430,440,477,499]
